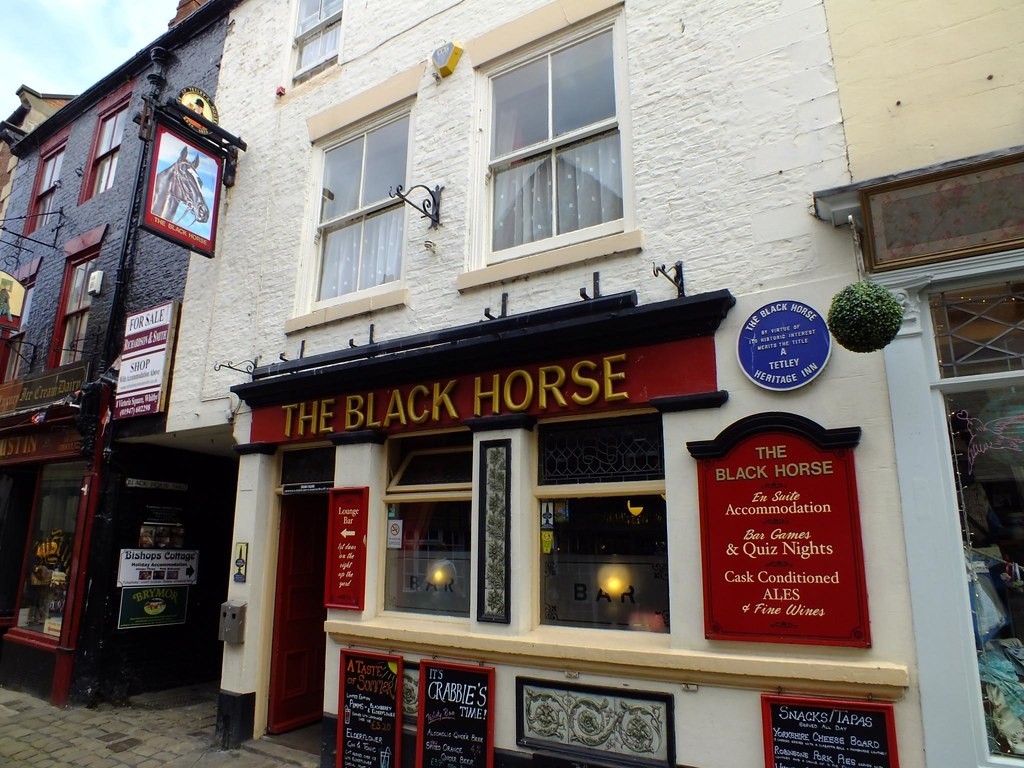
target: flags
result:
[31,411,46,425]
[102,409,112,437]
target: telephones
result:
[761,695,900,768]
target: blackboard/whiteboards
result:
[416,657,495,768]
[337,648,403,768]
